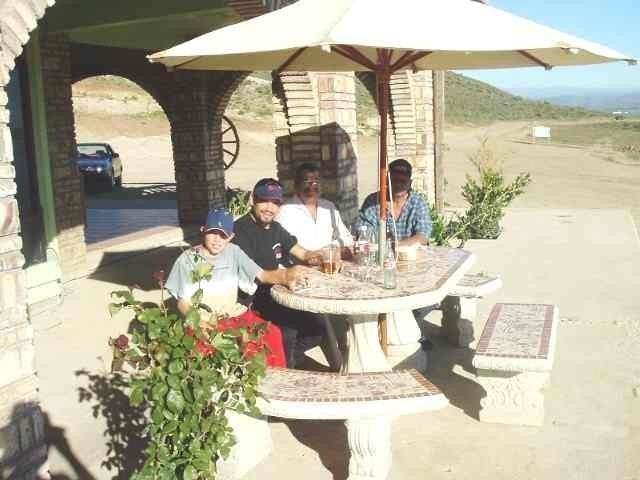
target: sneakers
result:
[420,336,434,350]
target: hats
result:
[204,207,233,237]
[389,159,411,180]
[253,179,283,205]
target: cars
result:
[74,141,125,193]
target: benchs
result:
[471,302,559,425]
[438,271,502,347]
[208,365,448,479]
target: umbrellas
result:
[147,0,640,356]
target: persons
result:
[229,177,344,372]
[272,164,354,265]
[353,159,434,249]
[163,209,308,369]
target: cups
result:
[318,246,338,275]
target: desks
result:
[271,244,474,371]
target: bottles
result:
[383,231,397,290]
[357,225,370,258]
